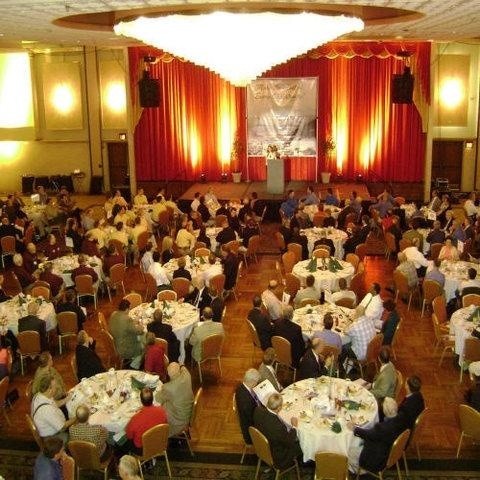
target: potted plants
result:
[317,135,337,184]
[230,128,245,183]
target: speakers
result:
[138,79,160,107]
[390,74,415,104]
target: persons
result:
[33,440,66,480]
[69,404,114,463]
[153,362,195,435]
[29,378,77,445]
[115,388,165,470]
[399,376,424,445]
[349,397,406,474]
[116,455,141,480]
[265,145,276,166]
[253,393,304,469]
[236,369,266,445]
[2,184,479,415]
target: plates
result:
[67,367,161,427]
[281,376,372,432]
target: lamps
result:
[112,7,366,88]
